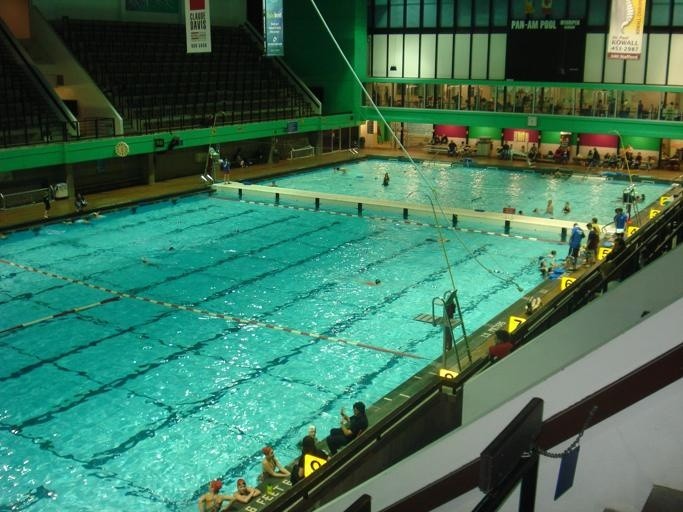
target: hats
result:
[237,479,245,486]
[308,425,316,432]
[263,447,272,455]
[210,480,222,490]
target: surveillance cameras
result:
[200,175,208,184]
[206,174,214,183]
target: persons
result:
[325,401,369,457]
[267,179,279,187]
[221,155,232,185]
[42,128,53,143]
[0,230,7,240]
[232,478,261,505]
[521,294,542,316]
[334,166,350,173]
[198,479,235,512]
[355,275,384,289]
[259,445,292,483]
[42,188,54,218]
[74,191,85,213]
[206,113,214,127]
[380,171,391,187]
[288,458,304,487]
[91,210,103,220]
[485,328,516,361]
[299,425,330,462]
[511,182,656,284]
[423,234,451,245]
[235,150,248,168]
[60,219,92,225]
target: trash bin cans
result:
[359,137,365,148]
[55,183,69,200]
